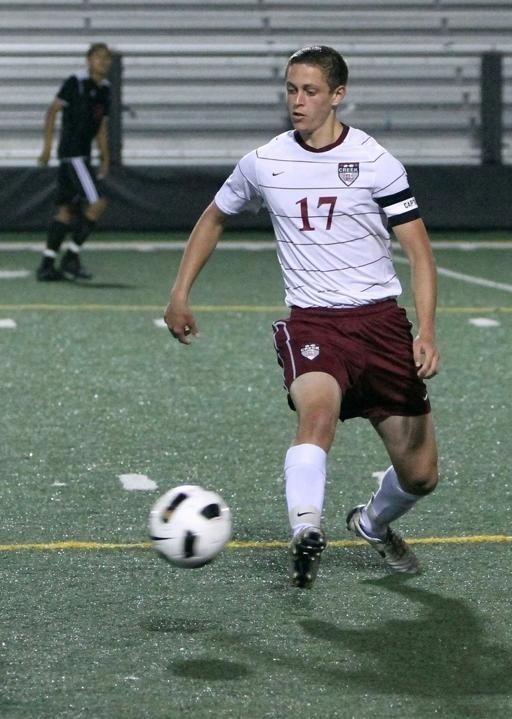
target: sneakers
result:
[346,505,419,574]
[37,255,91,281]
[289,527,327,589]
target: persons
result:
[163,46,441,590]
[37,44,112,281]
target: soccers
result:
[148,486,232,569]
